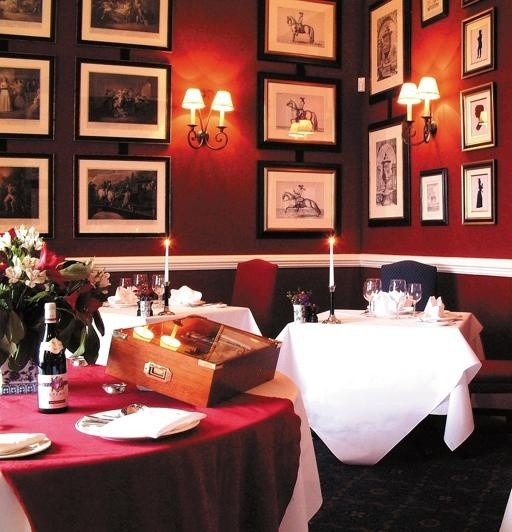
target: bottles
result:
[37,303,68,414]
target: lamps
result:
[398,77,440,145]
[180,87,233,151]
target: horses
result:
[98,189,120,207]
[282,192,321,217]
[286,15,314,44]
[286,99,317,132]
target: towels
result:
[170,286,203,303]
[0,430,45,457]
[425,295,444,318]
[371,290,411,311]
[101,406,209,438]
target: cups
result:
[306,306,318,322]
[168,298,186,314]
[120,278,131,292]
[136,300,166,317]
[133,327,153,342]
[160,336,181,352]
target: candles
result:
[327,236,335,288]
[163,239,170,280]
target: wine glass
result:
[389,279,407,320]
[408,283,422,318]
[152,274,165,305]
[363,278,383,318]
[131,274,149,301]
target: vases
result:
[1,358,40,395]
[139,300,152,317]
[291,304,307,323]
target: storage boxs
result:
[108,315,282,409]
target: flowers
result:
[285,288,315,305]
[0,226,117,371]
[133,284,155,301]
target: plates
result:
[74,409,200,442]
[110,304,138,307]
[189,300,206,307]
[434,317,463,321]
[0,434,51,459]
[400,306,417,314]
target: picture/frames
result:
[460,81,496,151]
[257,72,341,151]
[460,7,496,80]
[460,159,496,225]
[369,1,407,104]
[75,56,171,145]
[421,0,448,27]
[367,114,411,227]
[1,52,56,141]
[256,160,342,238]
[419,168,448,226]
[0,152,55,237]
[75,0,173,52]
[256,0,342,68]
[1,0,57,44]
[72,154,171,238]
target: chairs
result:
[468,360,512,395]
[230,259,279,337]
[379,260,437,314]
